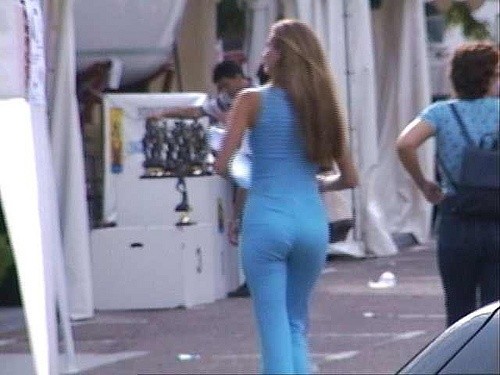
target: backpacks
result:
[435,102,500,215]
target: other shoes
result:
[227,282,251,298]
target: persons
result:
[156,56,271,298]
[215,18,360,375]
[395,43,499,328]
